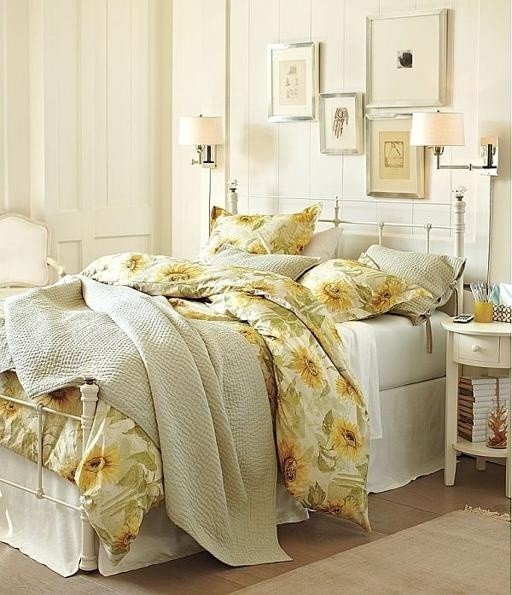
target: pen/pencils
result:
[470,280,496,303]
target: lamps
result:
[177,113,225,169]
[408,109,500,173]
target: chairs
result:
[0,209,66,299]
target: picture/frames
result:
[365,8,447,109]
[364,112,427,200]
[265,40,320,123]
[319,90,365,157]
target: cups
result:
[474,301,493,323]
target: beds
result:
[0,175,466,578]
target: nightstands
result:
[440,314,512,498]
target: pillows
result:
[197,201,467,354]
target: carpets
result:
[229,504,511,595]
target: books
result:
[459,376,511,443]
